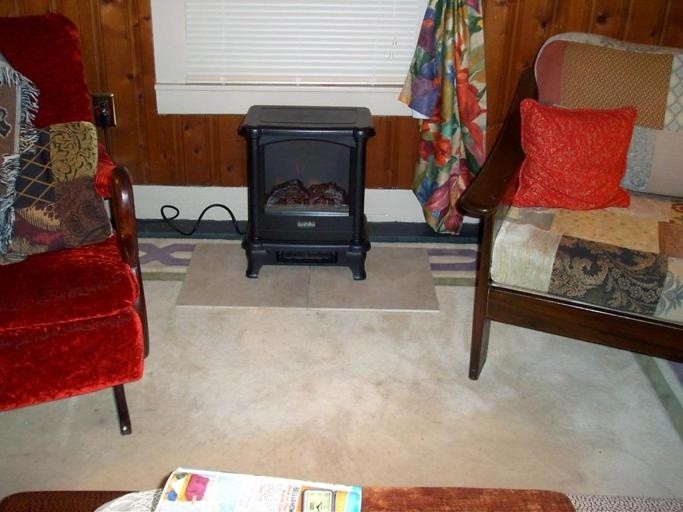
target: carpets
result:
[175,243,439,315]
[568,494,683,512]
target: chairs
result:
[0,54,111,267]
[0,13,161,436]
[454,33,683,382]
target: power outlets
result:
[90,94,116,128]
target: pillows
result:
[506,97,637,211]
